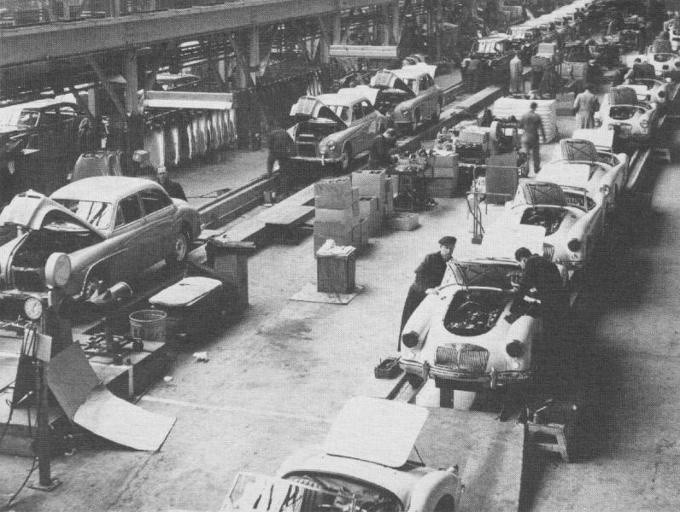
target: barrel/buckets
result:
[130,308,168,345]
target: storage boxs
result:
[314,168,399,257]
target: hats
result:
[438,236,457,245]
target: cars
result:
[0,0,680,285]
[222,398,465,512]
[0,176,200,302]
[409,223,552,390]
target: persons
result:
[266,123,294,177]
[397,235,456,351]
[509,50,523,93]
[465,59,480,92]
[155,166,187,200]
[639,21,647,54]
[571,82,600,128]
[518,102,547,173]
[611,58,641,86]
[510,247,570,320]
[367,128,398,169]
